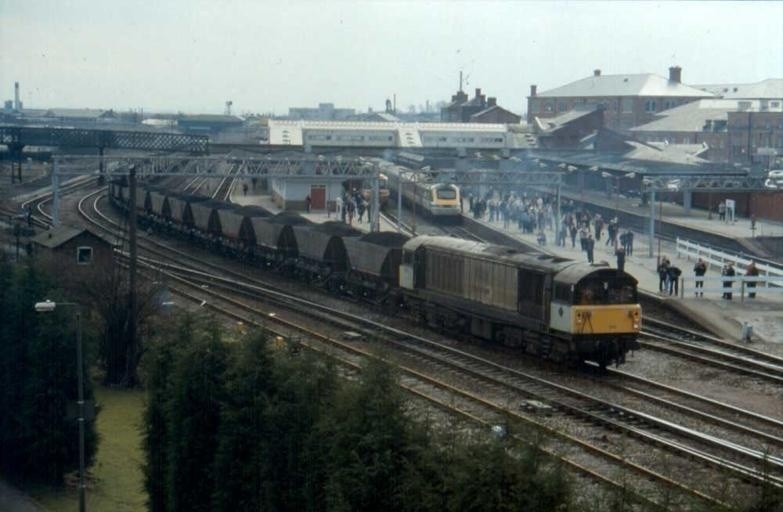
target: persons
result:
[457,185,633,272]
[717,200,726,221]
[303,193,312,213]
[692,257,706,298]
[656,261,670,292]
[743,262,756,297]
[332,186,370,227]
[724,262,735,300]
[720,264,727,298]
[750,260,758,297]
[666,263,681,297]
[661,253,671,288]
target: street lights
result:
[34,300,87,512]
[643,177,681,274]
